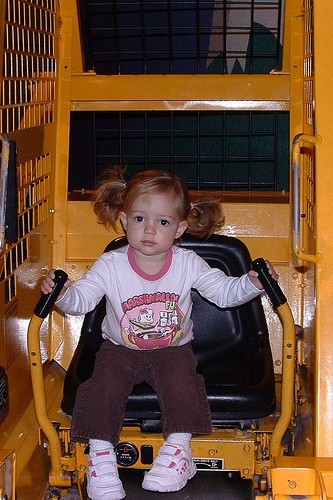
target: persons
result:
[40,165,280,500]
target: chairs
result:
[60,234,277,420]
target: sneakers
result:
[142,442,200,492]
[85,448,127,499]
